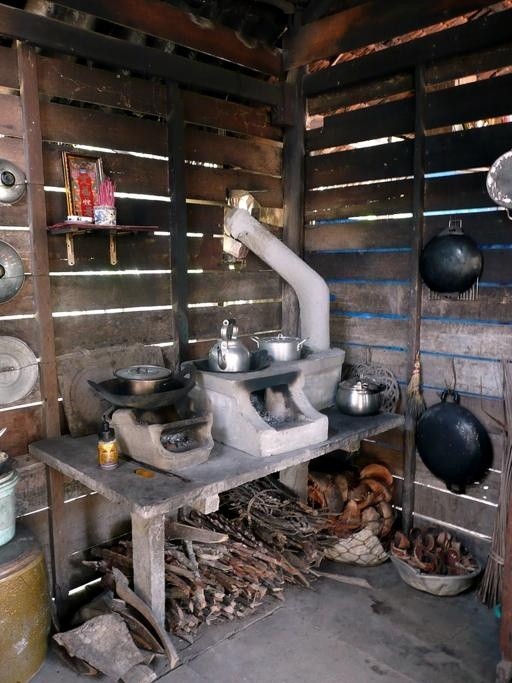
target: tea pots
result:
[207,317,251,373]
[335,374,387,416]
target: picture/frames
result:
[61,150,109,224]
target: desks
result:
[29,415,422,642]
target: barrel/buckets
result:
[0,470,18,546]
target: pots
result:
[415,388,495,495]
[113,363,174,396]
[86,364,197,411]
[418,219,483,302]
[250,331,310,362]
